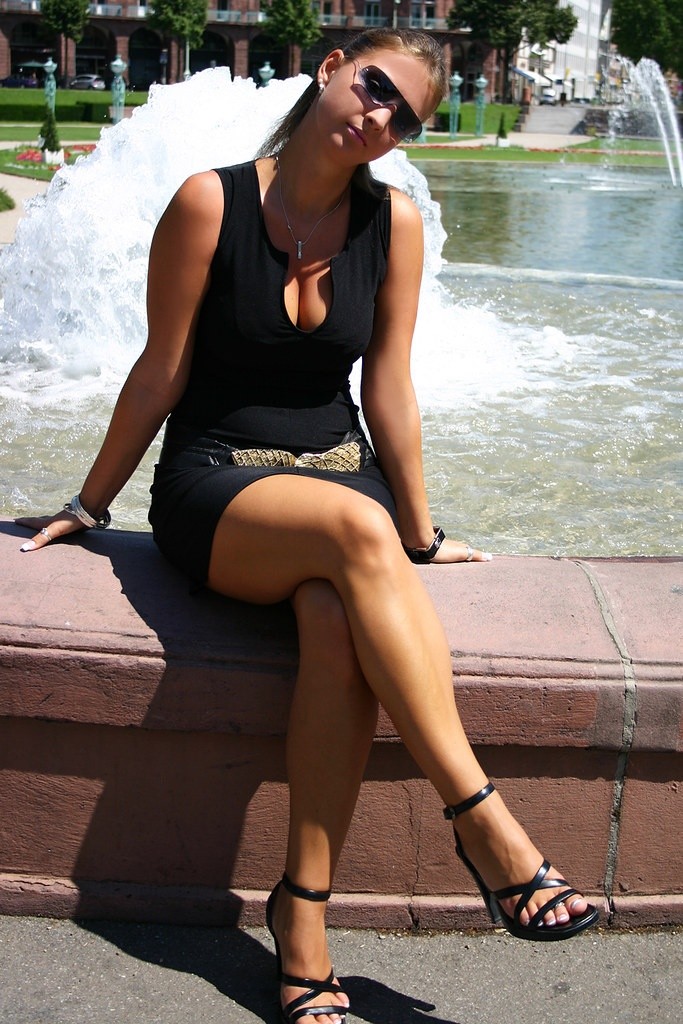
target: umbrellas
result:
[17,59,46,67]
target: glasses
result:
[345,55,424,143]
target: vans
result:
[539,87,558,107]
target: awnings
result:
[510,66,553,86]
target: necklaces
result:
[276,150,346,259]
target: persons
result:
[15,68,37,88]
[13,29,598,1024]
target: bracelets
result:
[63,493,112,529]
[402,525,446,564]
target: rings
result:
[467,545,473,562]
[41,528,52,542]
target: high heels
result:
[266,869,350,1024]
[442,782,601,942]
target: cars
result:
[69,73,111,91]
[0,73,39,88]
